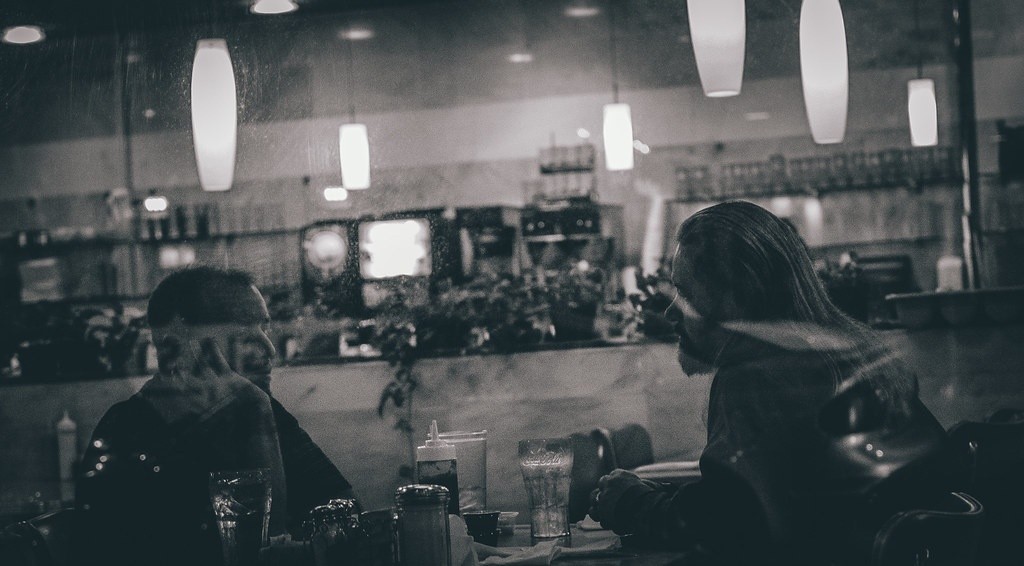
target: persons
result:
[75,266,363,566]
[588,200,949,566]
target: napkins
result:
[449,514,622,565]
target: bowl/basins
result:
[498,512,519,534]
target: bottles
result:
[57,410,77,480]
[416,420,460,516]
[395,483,451,566]
[310,499,394,566]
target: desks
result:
[258,524,702,566]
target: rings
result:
[596,491,602,503]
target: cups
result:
[517,437,574,538]
[426,430,488,512]
[463,512,500,547]
[208,468,273,545]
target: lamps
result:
[337,27,370,189]
[908,0,938,146]
[800,0,849,145]
[191,1,236,192]
[687,0,746,96]
[602,1,634,172]
